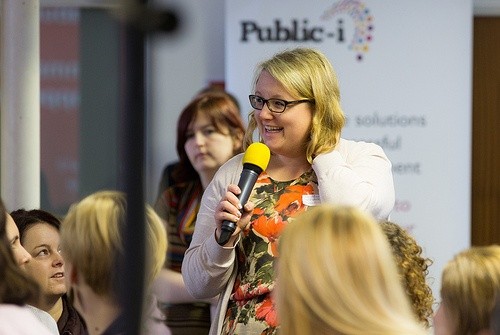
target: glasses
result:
[249,95,316,113]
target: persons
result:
[61,190,174,335]
[153,87,246,335]
[434,244,500,335]
[182,48,396,335]
[9,208,87,335]
[0,198,61,335]
[276,203,435,335]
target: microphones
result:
[218,142,270,246]
